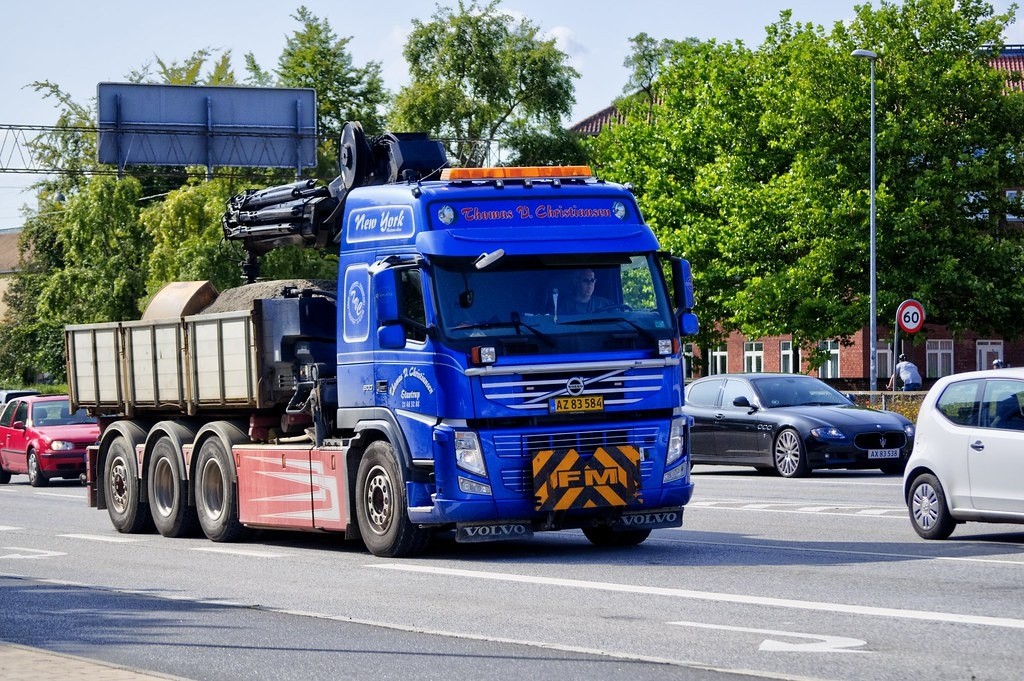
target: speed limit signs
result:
[898,299,927,334]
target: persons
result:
[553,269,622,314]
[992,359,1004,369]
[886,354,922,391]
[994,390,1024,430]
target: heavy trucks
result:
[63,165,698,563]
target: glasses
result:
[581,278,596,283]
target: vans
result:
[0,388,41,416]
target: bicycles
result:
[885,384,903,391]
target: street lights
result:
[851,47,879,405]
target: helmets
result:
[899,354,909,361]
[993,359,1003,367]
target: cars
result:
[0,395,105,487]
[902,367,1024,541]
[682,371,916,479]
